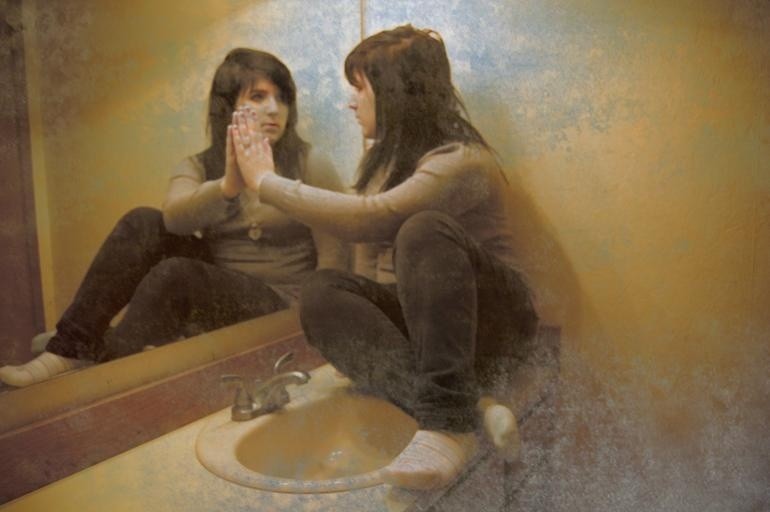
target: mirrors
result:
[0,0,367,433]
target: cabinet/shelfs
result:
[509,396,555,511]
[430,450,512,511]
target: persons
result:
[0,46,350,393]
[231,22,540,493]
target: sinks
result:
[195,383,420,495]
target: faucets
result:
[250,368,312,409]
